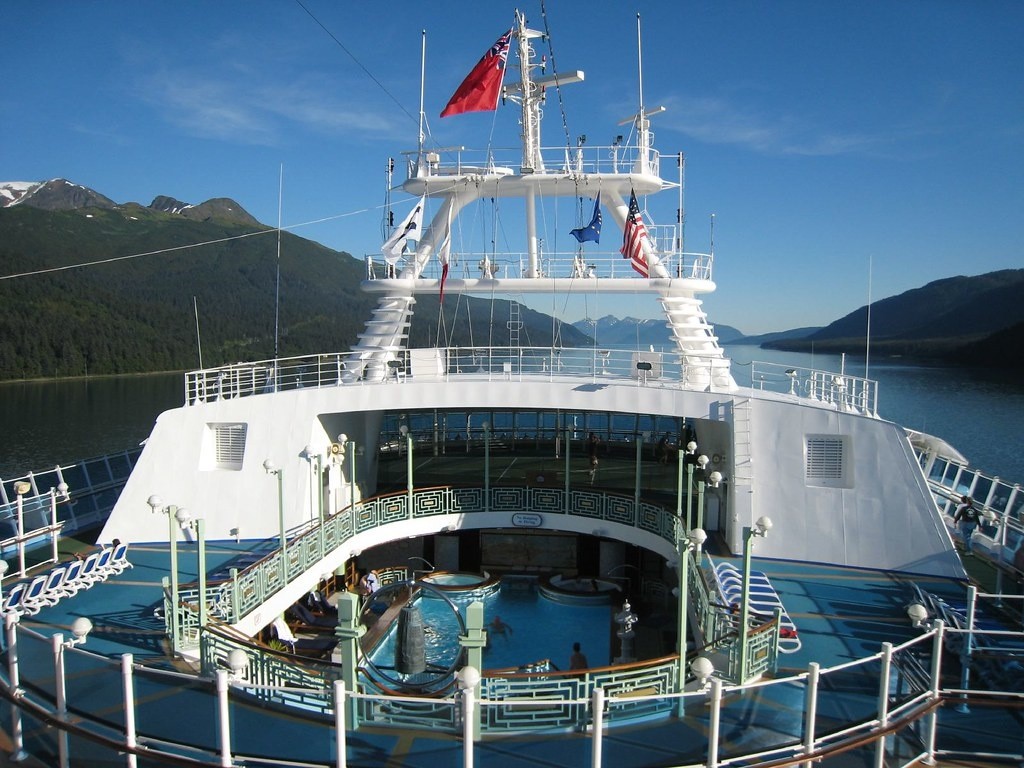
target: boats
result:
[0,8,1024,768]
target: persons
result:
[73,539,122,562]
[586,433,600,485]
[568,643,588,680]
[660,431,672,466]
[493,616,501,623]
[955,496,970,549]
[954,498,982,557]
[366,571,381,600]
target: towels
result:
[367,572,378,600]
[270,617,299,643]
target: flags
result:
[570,189,600,243]
[620,188,647,276]
[380,192,425,266]
[441,27,512,117]
[438,197,452,301]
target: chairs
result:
[0,542,133,621]
[705,550,802,654]
[258,590,337,667]
[903,580,1024,657]
